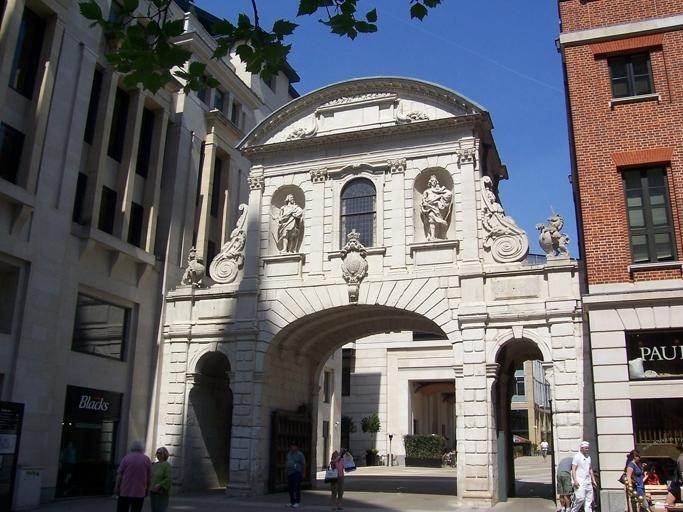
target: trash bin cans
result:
[12,465,45,511]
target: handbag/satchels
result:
[618,472,637,489]
[324,461,338,484]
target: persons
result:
[284,437,305,506]
[664,449,682,503]
[625,450,652,511]
[150,446,171,512]
[330,450,345,510]
[114,439,150,510]
[540,438,547,461]
[570,440,598,511]
[418,174,453,243]
[642,463,659,484]
[556,455,575,510]
[270,193,304,255]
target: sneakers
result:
[560,506,571,512]
[285,502,302,509]
[332,507,344,512]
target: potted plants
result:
[360,412,381,466]
[401,433,448,468]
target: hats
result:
[579,441,590,448]
[287,440,299,447]
